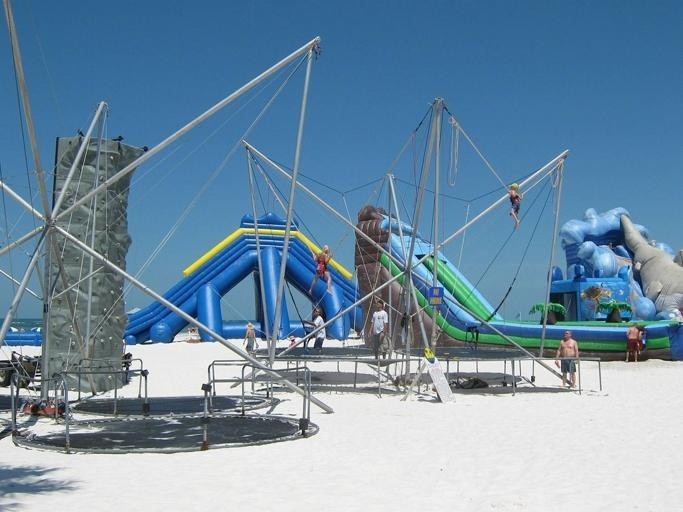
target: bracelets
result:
[381,331,385,333]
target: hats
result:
[509,183,519,189]
[289,336,296,341]
[247,323,253,329]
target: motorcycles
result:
[0,350,39,389]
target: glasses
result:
[377,300,383,309]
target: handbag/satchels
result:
[457,375,488,389]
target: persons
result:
[308,245,334,296]
[17,401,65,416]
[626,322,638,363]
[367,300,388,358]
[507,182,523,230]
[629,327,648,357]
[286,336,297,348]
[554,329,579,387]
[301,308,326,348]
[242,322,257,353]
